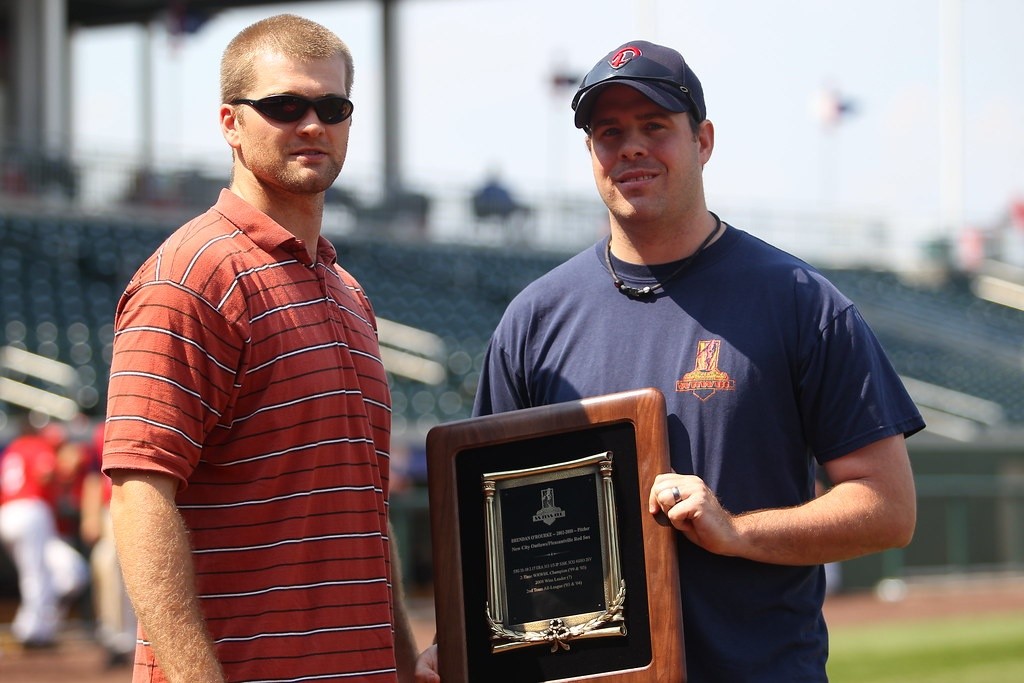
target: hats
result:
[573,41,707,136]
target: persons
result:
[0,417,135,672]
[413,38,927,682]
[100,13,420,683]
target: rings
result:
[671,486,683,504]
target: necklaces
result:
[604,210,721,297]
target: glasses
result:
[230,93,354,125]
[571,55,701,136]
